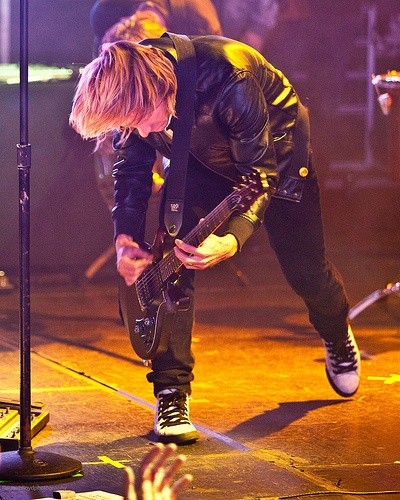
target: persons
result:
[121,442,194,500]
[65,0,361,442]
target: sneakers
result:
[321,318,361,397]
[152,387,201,444]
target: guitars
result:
[118,166,270,361]
